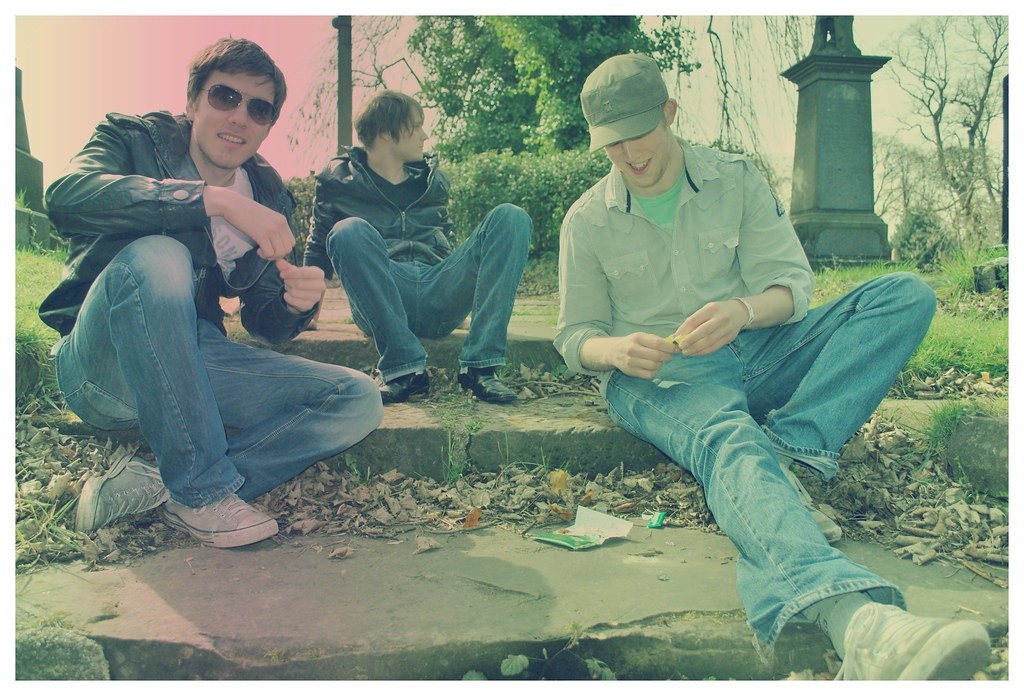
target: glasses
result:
[200,84,276,126]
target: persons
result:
[554,52,992,679]
[303,90,534,402]
[37,36,383,548]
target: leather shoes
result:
[458,364,517,401]
[380,367,430,403]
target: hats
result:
[581,54,669,153]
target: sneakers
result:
[780,462,843,541]
[833,601,992,681]
[77,454,170,532]
[162,485,280,548]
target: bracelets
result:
[731,297,754,329]
